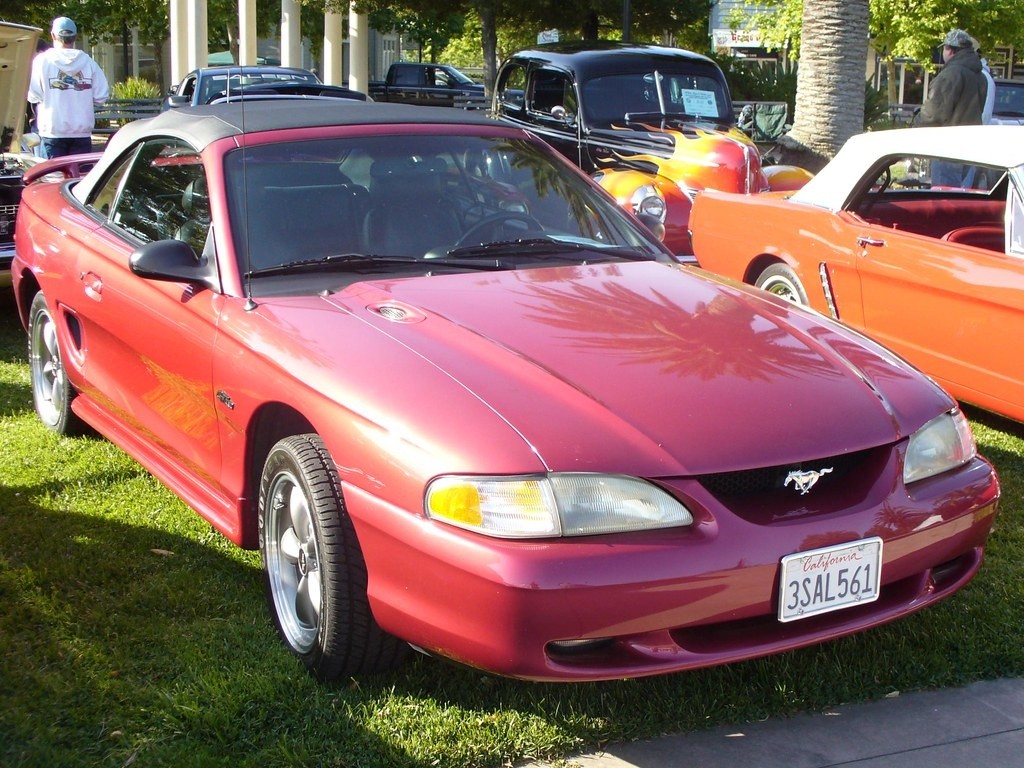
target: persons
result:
[25,17,109,172]
[915,30,997,188]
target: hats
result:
[50,15,78,39]
[970,36,981,52]
[936,28,970,49]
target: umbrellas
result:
[207,51,282,66]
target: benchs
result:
[861,200,1006,254]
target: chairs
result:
[175,174,279,269]
[365,160,459,260]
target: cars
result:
[685,123,1024,424]
[159,39,817,267]
[9,91,1002,693]
